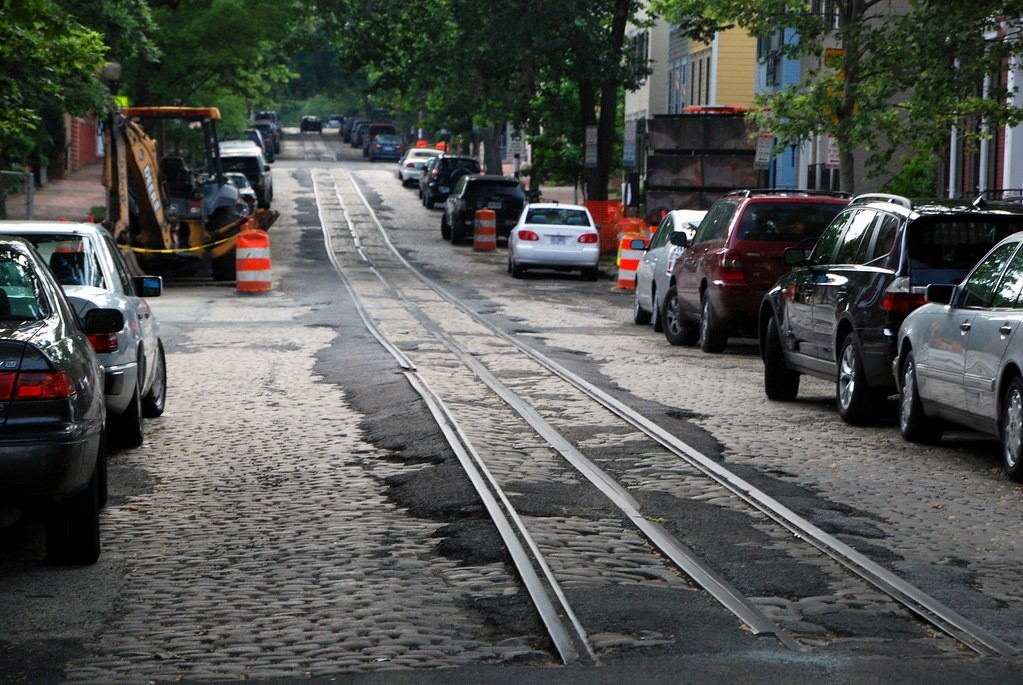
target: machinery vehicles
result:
[95,101,279,283]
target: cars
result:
[299,115,322,134]
[204,107,283,214]
[0,233,125,568]
[327,113,344,129]
[366,133,406,162]
[506,202,602,281]
[890,230,1023,483]
[0,216,168,450]
[396,147,443,188]
[632,208,710,332]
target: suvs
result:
[439,174,528,244]
[756,187,1023,426]
[417,155,482,210]
[338,117,395,157]
[661,186,860,353]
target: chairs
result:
[567,217,581,224]
[50,253,93,286]
[1,288,11,318]
[533,215,545,223]
